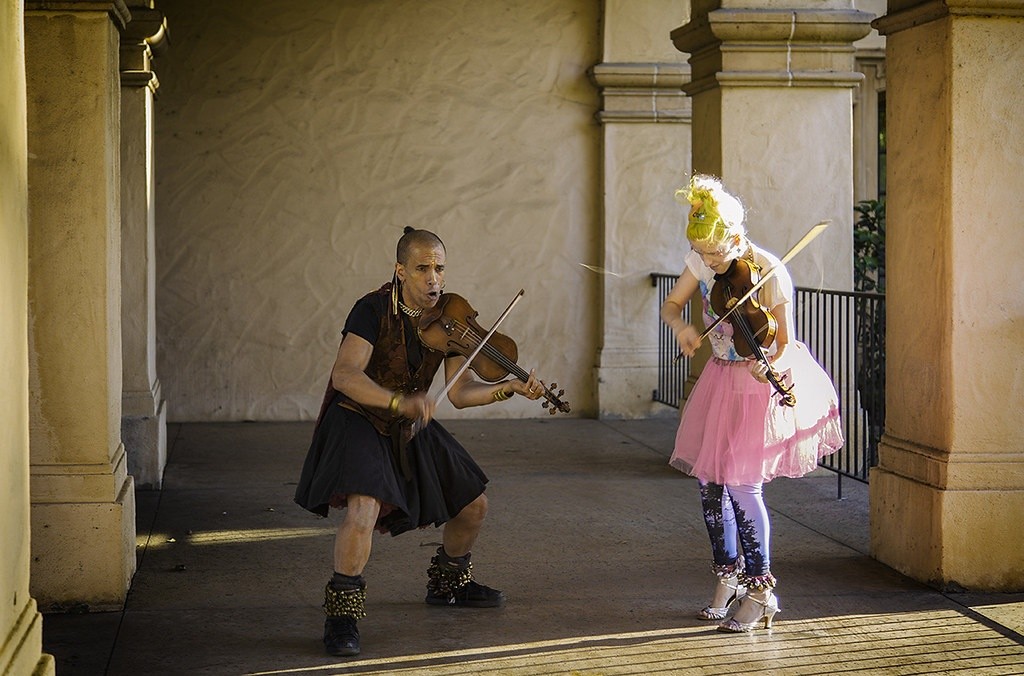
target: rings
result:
[529,388,535,395]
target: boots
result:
[323,574,367,656]
[424,547,503,608]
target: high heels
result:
[716,591,779,632]
[696,573,746,620]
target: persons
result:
[293,226,545,659]
[660,174,845,633]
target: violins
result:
[711,259,796,408]
[417,293,571,415]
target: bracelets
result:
[387,393,403,413]
[493,389,515,401]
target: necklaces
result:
[747,242,755,263]
[397,301,423,318]
